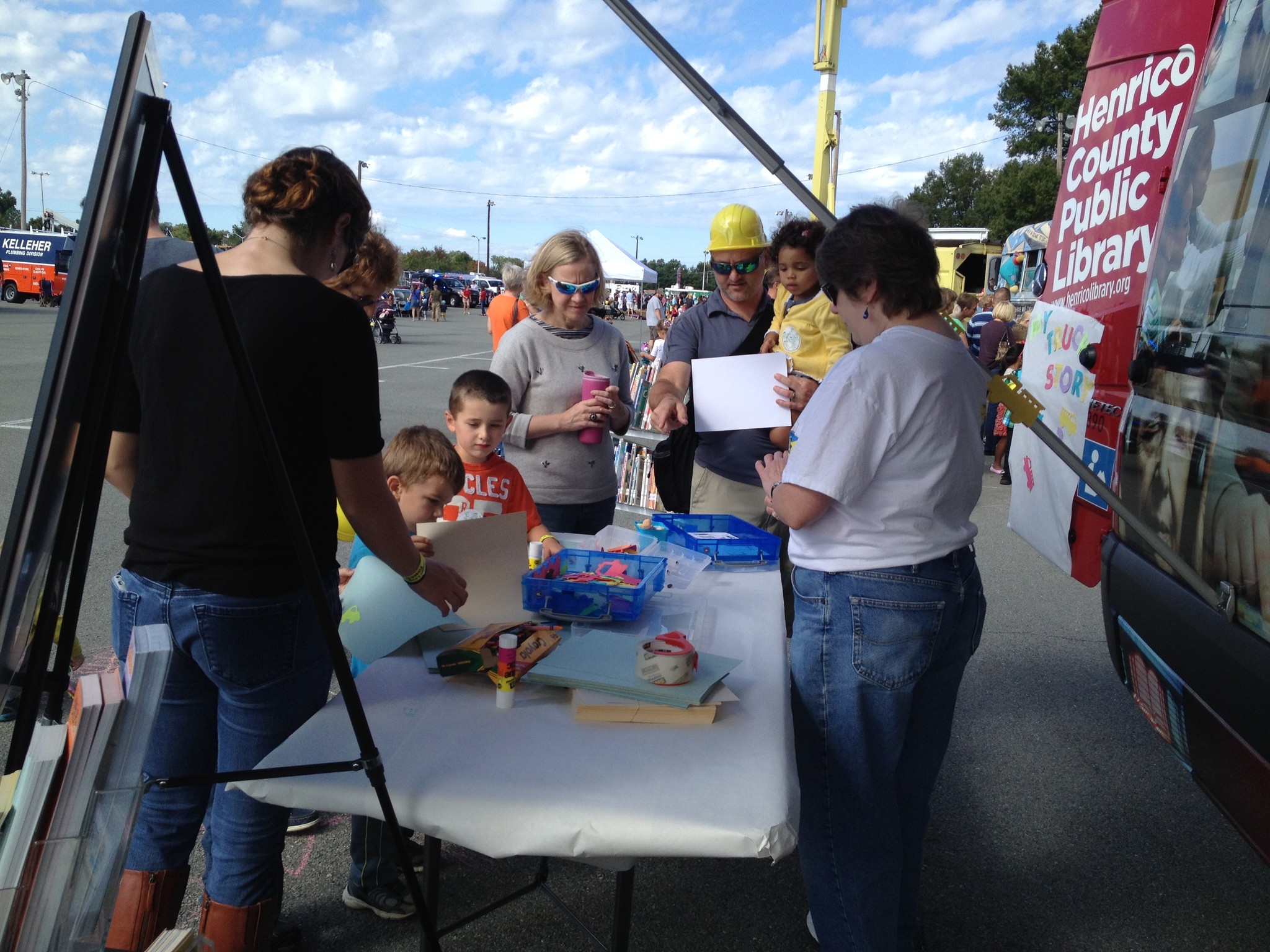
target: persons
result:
[1126,0,1270,619]
[754,204,989,952]
[102,146,470,952]
[139,195,221,282]
[285,204,1033,918]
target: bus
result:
[665,284,713,306]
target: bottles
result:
[579,367,609,444]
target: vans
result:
[1016,1,1270,857]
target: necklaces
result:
[241,236,297,261]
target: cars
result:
[390,269,507,316]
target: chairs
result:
[399,301,410,318]
[393,301,400,318]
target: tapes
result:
[636,637,695,686]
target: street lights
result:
[486,199,496,276]
[357,160,368,187]
[0,70,31,232]
[30,171,51,230]
[472,235,486,273]
[630,235,644,284]
[702,250,709,291]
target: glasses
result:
[820,279,840,306]
[346,284,380,307]
[710,249,766,275]
[547,274,600,294]
[339,225,358,275]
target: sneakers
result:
[341,878,416,919]
[407,839,424,871]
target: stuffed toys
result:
[999,252,1026,294]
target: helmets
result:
[708,205,772,251]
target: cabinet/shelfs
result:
[0,774,145,952]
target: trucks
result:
[0,231,79,303]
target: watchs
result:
[771,480,783,498]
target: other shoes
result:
[463,311,486,316]
[1000,477,1011,485]
[984,450,995,456]
[412,315,446,321]
[285,806,321,833]
[990,464,1005,474]
[806,910,819,942]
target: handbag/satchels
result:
[647,424,698,514]
[404,302,411,311]
[995,329,1011,361]
[419,301,421,306]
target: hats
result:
[433,285,438,288]
[441,300,445,304]
[423,299,427,302]
[656,288,666,296]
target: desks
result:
[223,532,801,952]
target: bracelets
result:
[538,535,560,549]
[403,553,428,584]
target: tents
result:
[524,229,659,353]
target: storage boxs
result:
[483,513,781,624]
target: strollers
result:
[370,298,402,345]
[605,300,625,321]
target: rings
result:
[589,413,597,421]
[791,391,796,399]
[608,401,617,408]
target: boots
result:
[104,862,191,951]
[197,885,283,952]
